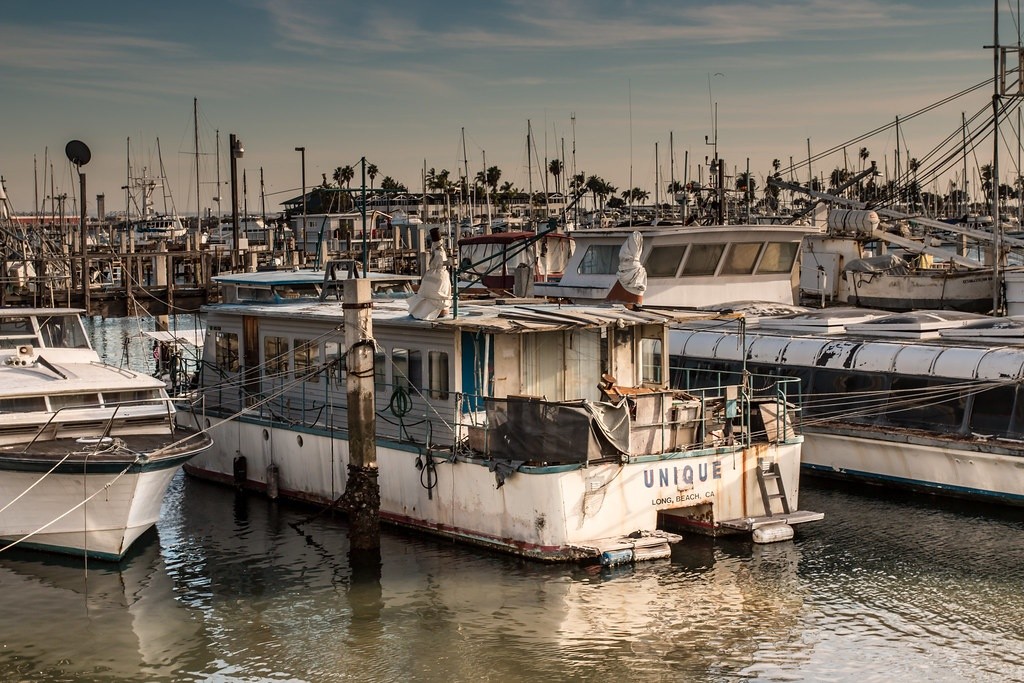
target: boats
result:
[0,144,215,560]
[140,133,826,577]
[13,0,1023,396]
[459,226,1023,511]
